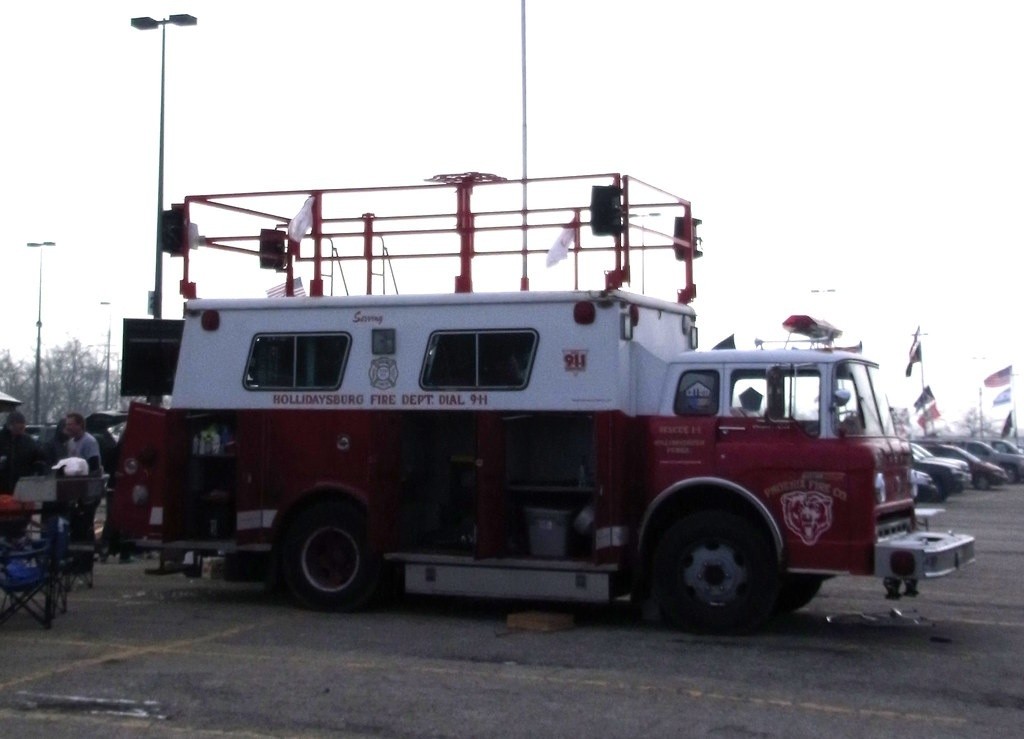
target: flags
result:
[904,327,1013,426]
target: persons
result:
[0,411,44,495]
[40,414,140,571]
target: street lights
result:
[130,9,196,320]
[26,240,56,425]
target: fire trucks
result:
[103,172,978,640]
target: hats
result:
[6,412,25,426]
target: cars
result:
[903,436,1024,503]
